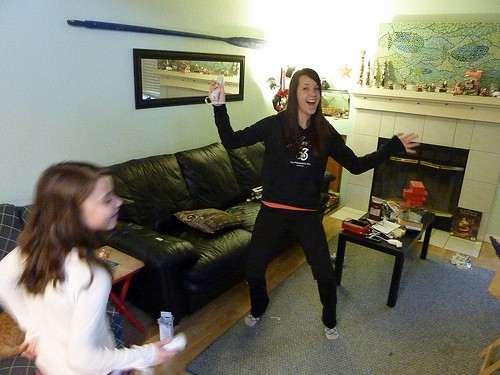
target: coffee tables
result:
[335,203,434,306]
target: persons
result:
[209,68,419,339]
[0,160,178,375]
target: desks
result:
[96,247,145,337]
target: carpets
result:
[184,232,500,375]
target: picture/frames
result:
[132,48,245,109]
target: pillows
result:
[173,208,246,234]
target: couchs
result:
[0,203,125,375]
[93,140,335,326]
